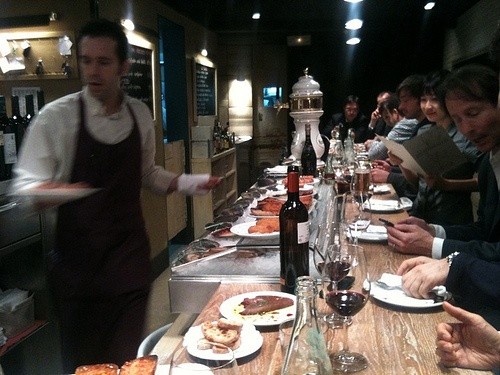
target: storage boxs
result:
[192,116,218,158]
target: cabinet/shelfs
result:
[191,147,238,241]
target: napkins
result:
[367,197,413,210]
[379,272,448,300]
[355,222,388,234]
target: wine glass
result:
[311,221,353,328]
[320,243,372,373]
[340,189,373,259]
[355,167,373,219]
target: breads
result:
[247,175,313,233]
[202,318,242,353]
[74,355,159,375]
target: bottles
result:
[301,124,317,177]
[282,275,333,375]
[324,130,348,174]
[340,137,360,167]
[0,89,44,178]
[279,165,310,293]
[213,121,235,155]
[325,153,335,185]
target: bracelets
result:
[447,251,460,264]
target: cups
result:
[168,341,240,375]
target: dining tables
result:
[151,151,494,375]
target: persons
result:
[325,65,500,371]
[10,19,223,375]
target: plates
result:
[347,224,389,242]
[363,200,404,213]
[7,188,104,200]
[219,291,297,327]
[186,323,265,361]
[354,144,390,193]
[229,178,320,240]
[369,272,452,309]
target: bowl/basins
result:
[137,323,174,359]
